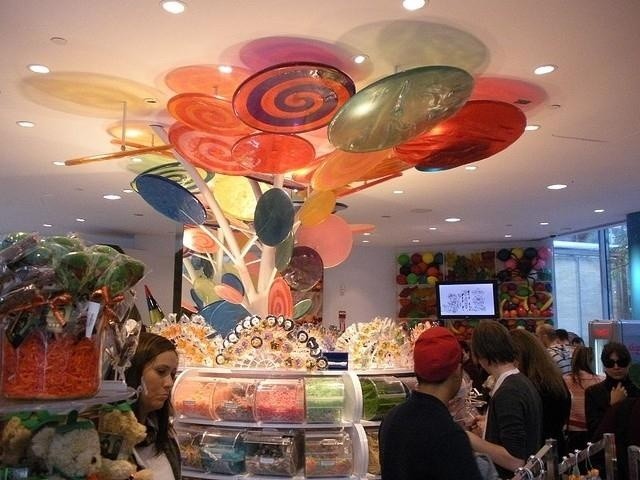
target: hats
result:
[414,326,462,381]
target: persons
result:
[104,332,182,480]
[378,326,483,480]
[454,319,640,480]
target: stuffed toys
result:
[0,400,148,480]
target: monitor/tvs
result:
[435,279,499,319]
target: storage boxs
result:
[1,313,106,400]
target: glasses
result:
[605,359,629,368]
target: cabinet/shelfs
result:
[396,246,557,336]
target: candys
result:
[0,60,528,369]
